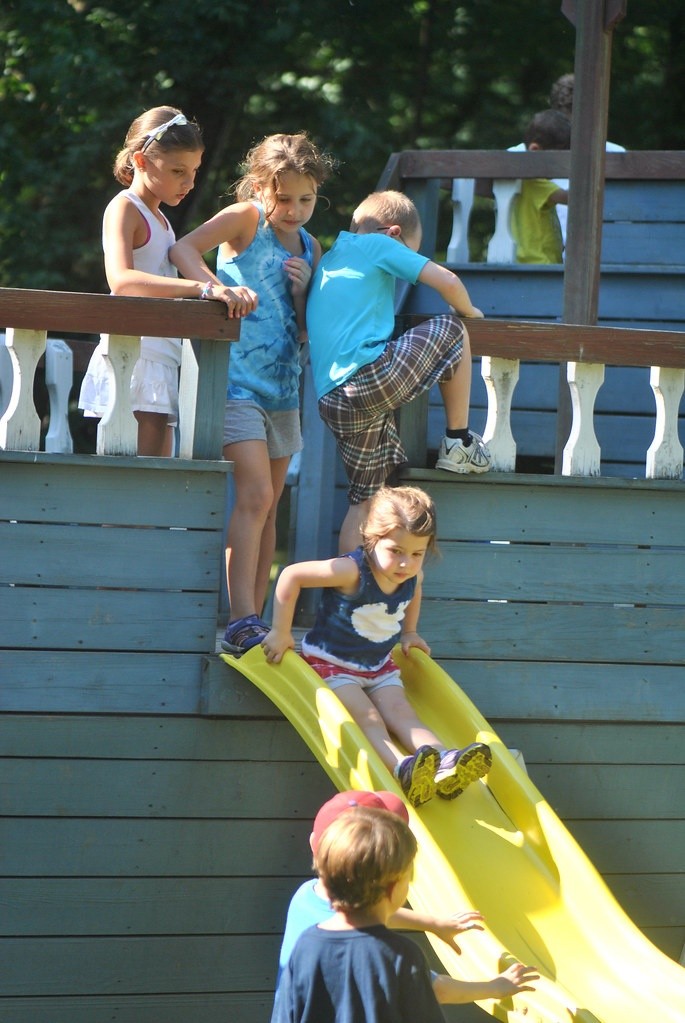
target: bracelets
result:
[200,282,216,298]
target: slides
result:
[223,644,685,1023]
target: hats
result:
[313,790,409,859]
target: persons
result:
[258,488,494,808]
[80,106,258,456]
[479,71,625,262]
[304,189,498,556]
[166,134,336,653]
[270,792,539,1023]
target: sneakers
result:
[399,745,438,808]
[222,615,271,652]
[435,430,494,474]
[436,743,492,800]
